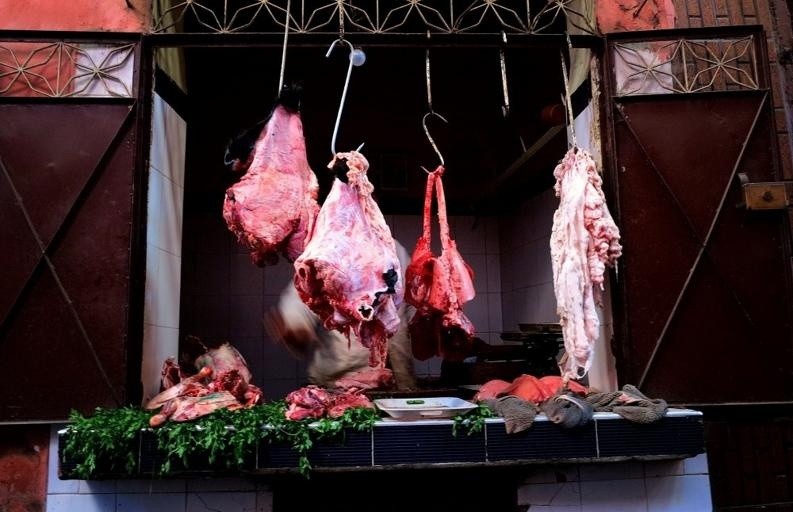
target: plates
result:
[375,395,480,422]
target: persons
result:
[267,210,413,399]
